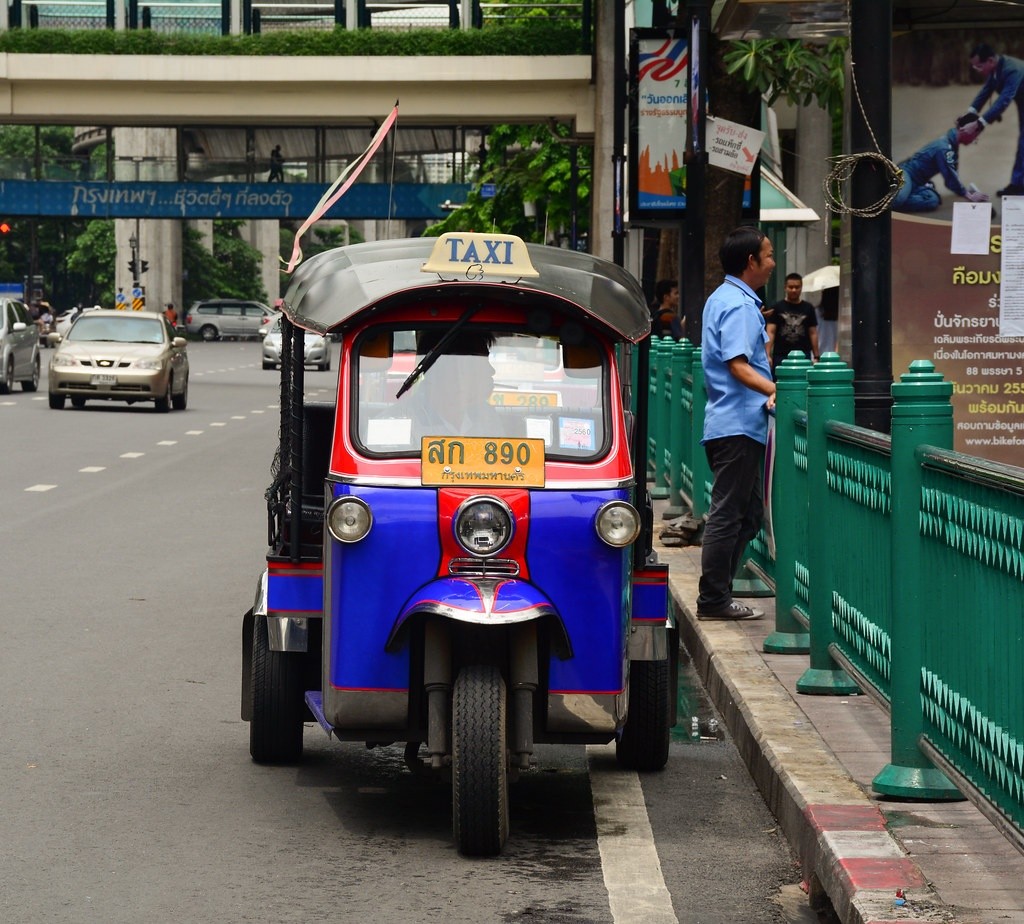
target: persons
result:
[162,303,178,332]
[889,42,1024,213]
[759,271,839,384]
[266,142,288,183]
[360,322,528,452]
[641,280,687,343]
[30,300,101,334]
[695,226,777,621]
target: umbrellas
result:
[798,265,841,292]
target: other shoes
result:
[996,184,1024,197]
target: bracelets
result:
[814,356,820,360]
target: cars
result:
[259,311,341,372]
[0,297,42,394]
[46,305,191,414]
[56,304,106,339]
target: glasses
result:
[972,60,989,71]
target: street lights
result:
[129,231,138,282]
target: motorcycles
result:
[236,228,684,857]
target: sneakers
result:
[697,601,765,620]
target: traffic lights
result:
[0,223,11,238]
[141,260,149,273]
[126,261,134,274]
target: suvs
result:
[186,299,276,341]
